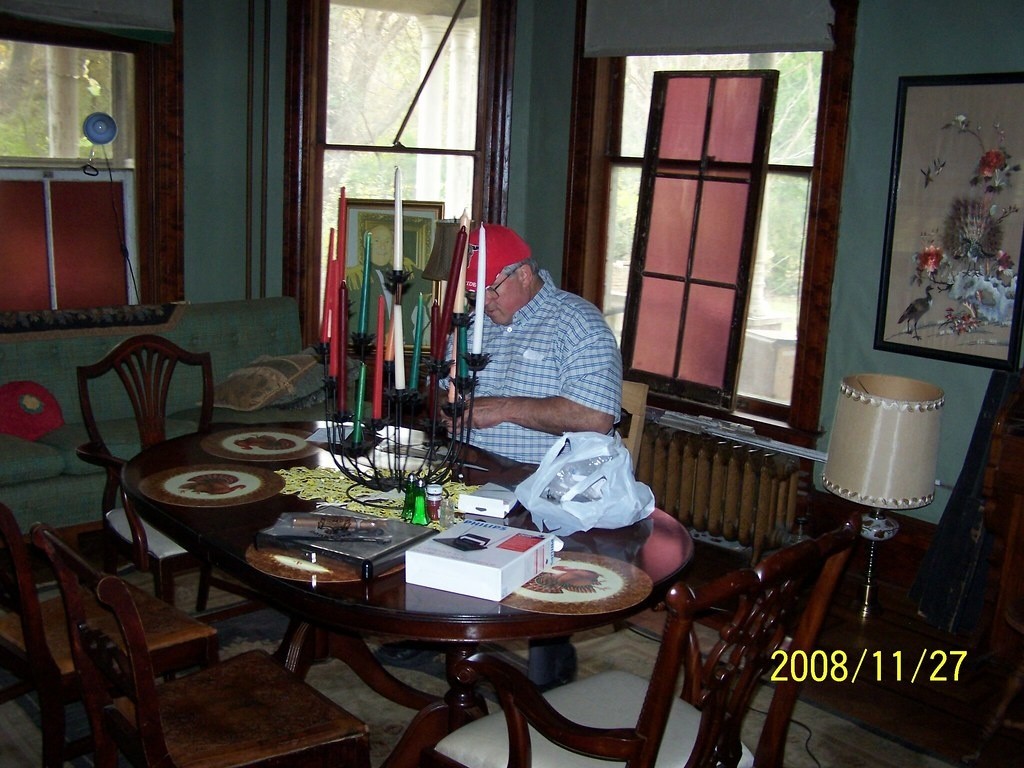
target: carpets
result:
[0,559,957,768]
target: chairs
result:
[621,380,652,478]
[27,523,372,768]
[74,333,223,606]
[426,511,864,768]
[0,502,228,768]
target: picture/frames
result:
[338,198,448,358]
[872,70,1024,375]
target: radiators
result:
[636,404,833,567]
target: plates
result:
[245,544,404,584]
[201,427,319,462]
[497,551,653,614]
[136,464,286,508]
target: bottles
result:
[439,498,455,528]
[400,474,414,520]
[427,484,443,521]
[410,479,432,526]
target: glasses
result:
[465,265,523,301]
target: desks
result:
[119,421,699,712]
[957,599,1024,768]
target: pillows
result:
[198,353,320,411]
[0,381,64,443]
[263,347,362,410]
[434,670,755,768]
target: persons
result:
[346,225,433,345]
[425,224,623,465]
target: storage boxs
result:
[404,519,554,605]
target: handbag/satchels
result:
[513,428,655,538]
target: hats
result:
[466,224,532,292]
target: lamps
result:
[819,372,948,624]
[78,110,144,304]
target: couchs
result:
[0,296,368,549]
